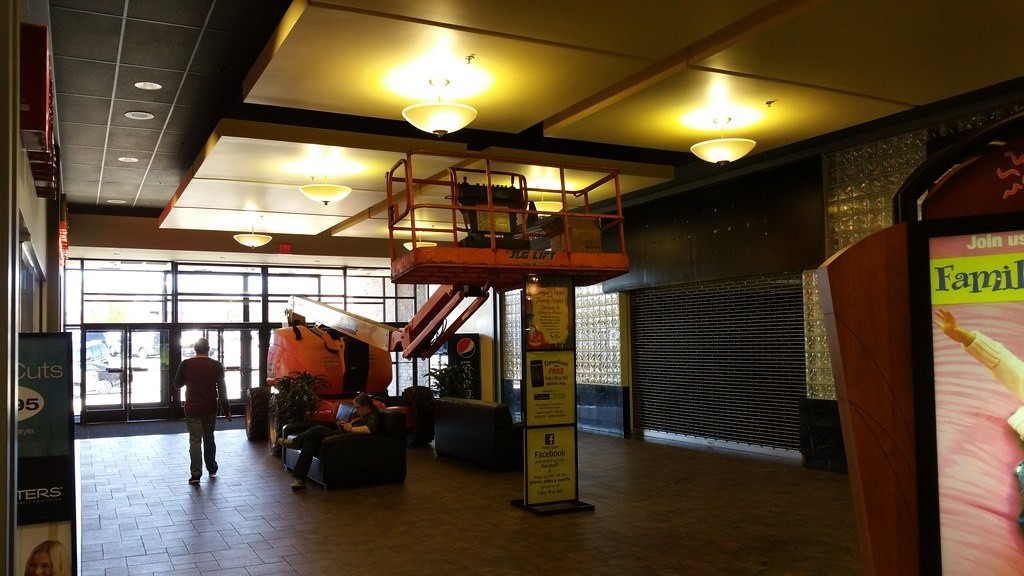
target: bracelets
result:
[349,427,353,433]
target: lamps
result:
[689,116,757,166]
[300,176,353,206]
[402,80,478,136]
[232,226,272,248]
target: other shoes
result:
[211,472,216,477]
[291,480,304,489]
[189,476,200,484]
[278,438,300,449]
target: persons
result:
[173,338,232,485]
[24,541,72,576]
[278,394,383,492]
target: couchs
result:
[281,409,408,493]
[433,396,524,473]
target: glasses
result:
[355,406,362,410]
